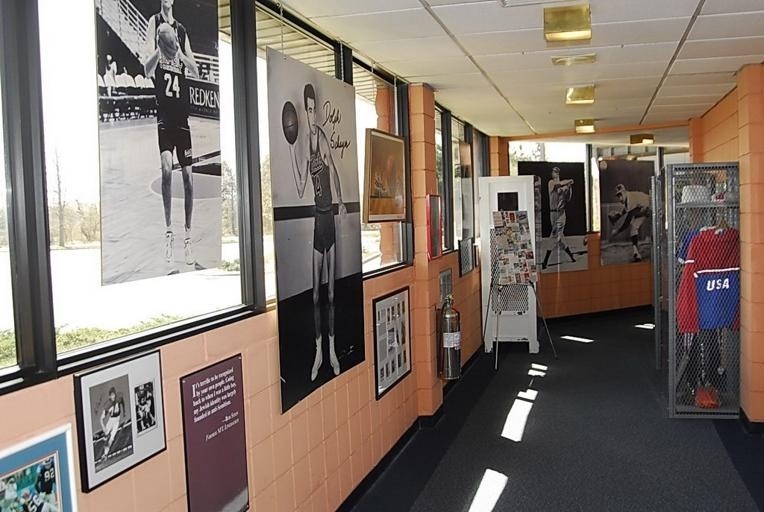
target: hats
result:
[552,167,561,173]
[675,185,718,205]
[614,184,625,194]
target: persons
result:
[541,167,576,270]
[144,0,200,265]
[99,387,127,461]
[288,83,346,381]
[135,386,156,430]
[607,184,652,262]
[0,458,58,512]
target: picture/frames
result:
[371,285,412,401]
[458,237,474,278]
[425,194,441,261]
[0,347,168,512]
[361,127,408,225]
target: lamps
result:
[541,4,596,106]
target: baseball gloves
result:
[608,209,623,225]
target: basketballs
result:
[283,102,299,145]
[157,22,177,59]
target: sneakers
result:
[163,227,180,274]
[183,225,196,265]
[311,335,323,381]
[632,246,641,260]
[329,334,340,375]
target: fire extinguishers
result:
[438,294,462,380]
